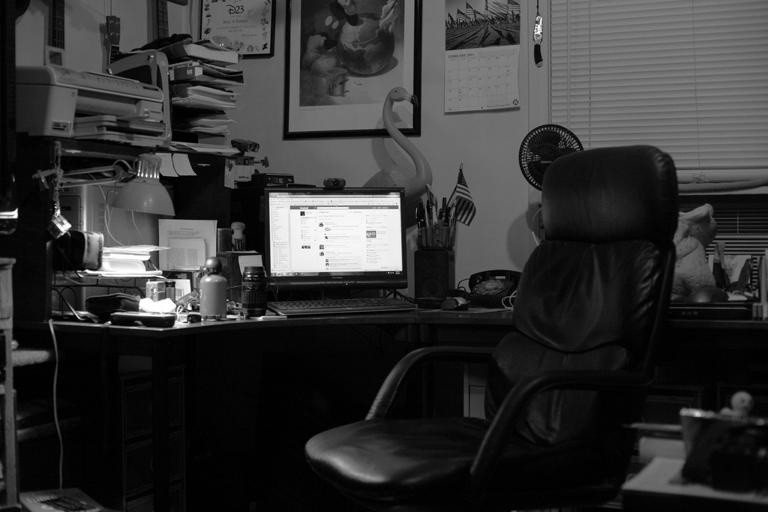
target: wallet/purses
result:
[86,293,139,314]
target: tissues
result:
[109,296,177,326]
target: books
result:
[157,38,244,155]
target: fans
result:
[521,124,584,191]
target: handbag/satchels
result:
[55,230,103,270]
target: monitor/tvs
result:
[262,187,409,290]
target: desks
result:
[1,134,768,512]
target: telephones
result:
[468,270,522,308]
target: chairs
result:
[302,144,680,511]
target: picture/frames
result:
[200,0,276,59]
[283,1,422,139]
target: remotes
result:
[110,310,177,328]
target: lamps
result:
[59,153,177,218]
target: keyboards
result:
[267,297,418,316]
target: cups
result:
[414,248,456,309]
[240,266,268,318]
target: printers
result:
[15,65,173,149]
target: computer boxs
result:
[230,174,293,274]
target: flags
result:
[454,170,477,228]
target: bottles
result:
[198,257,229,320]
[164,280,176,303]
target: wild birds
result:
[360,86,433,201]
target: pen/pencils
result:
[415,196,458,249]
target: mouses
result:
[440,296,469,311]
